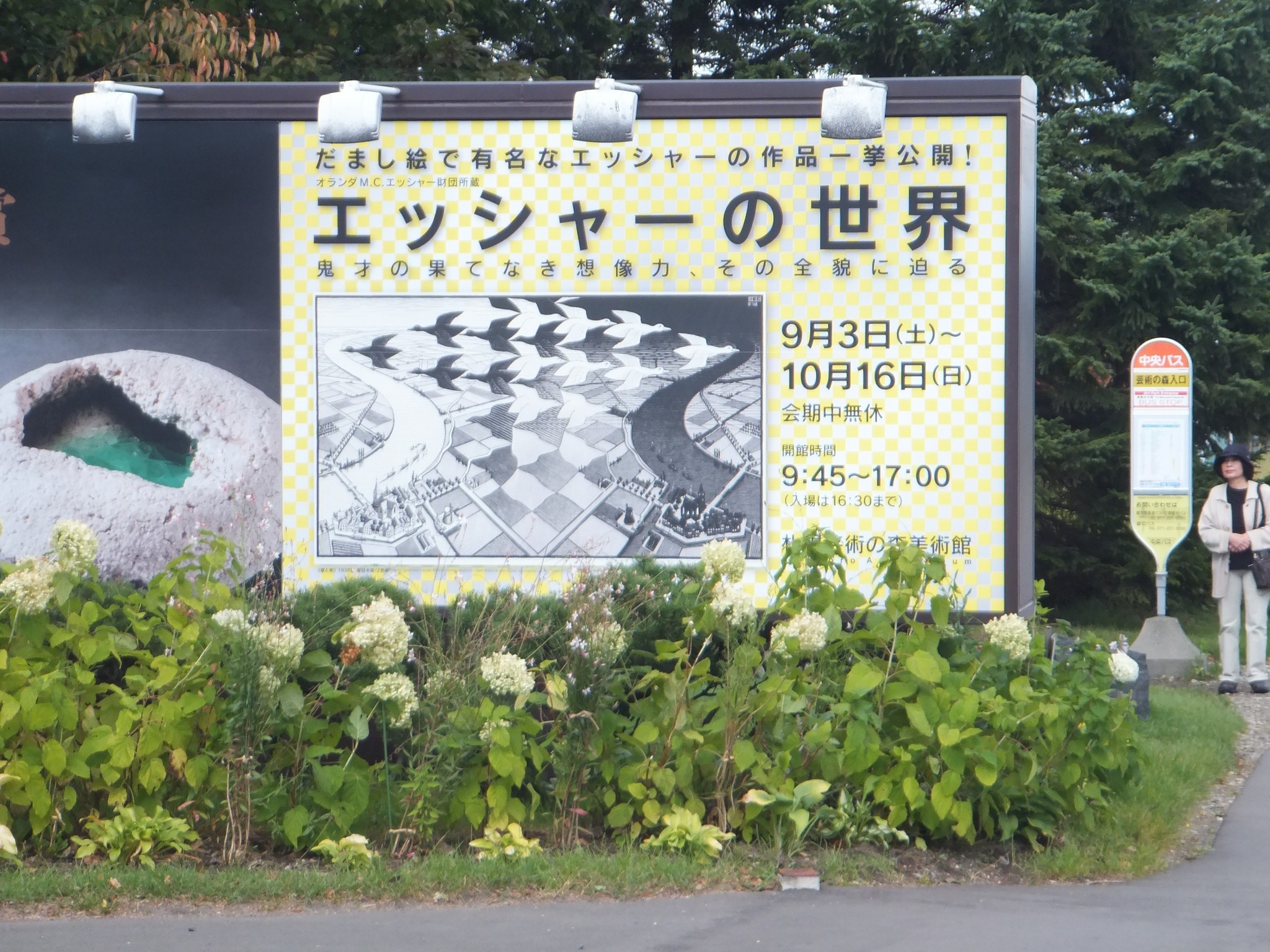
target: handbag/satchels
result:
[1253,550,1270,589]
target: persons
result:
[1198,444,1270,694]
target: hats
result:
[1213,443,1254,480]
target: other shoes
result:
[1251,681,1268,693]
[1218,681,1235,693]
